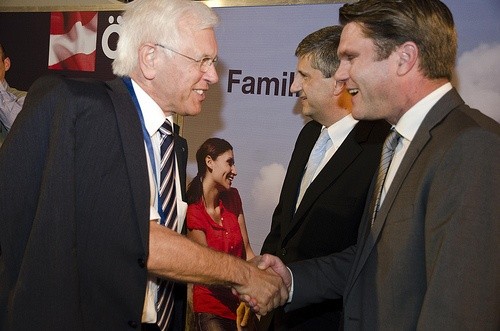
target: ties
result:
[294,129,330,214]
[368,128,401,229]
[156,118,178,331]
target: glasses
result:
[154,44,217,73]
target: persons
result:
[230,0,500,331]
[231,24,392,331]
[0,0,287,331]
[185,137,257,331]
